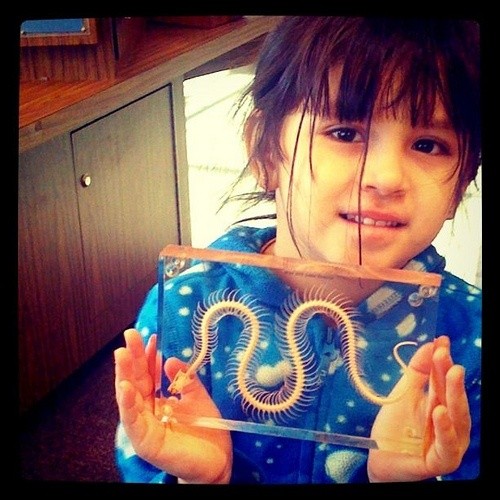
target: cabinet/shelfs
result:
[17,18,286,418]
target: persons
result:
[114,15,482,490]
